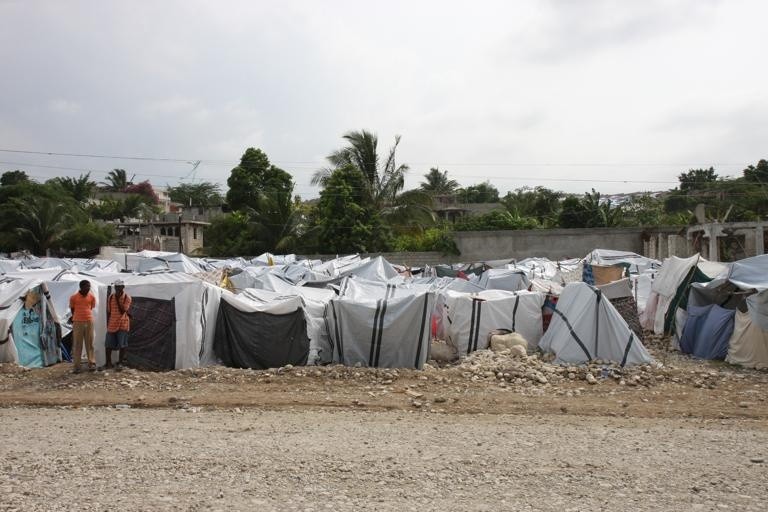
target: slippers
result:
[97,363,124,371]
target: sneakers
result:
[73,368,80,373]
[89,365,96,370]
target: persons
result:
[98,280,131,371]
[68,280,97,374]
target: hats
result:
[115,280,124,285]
